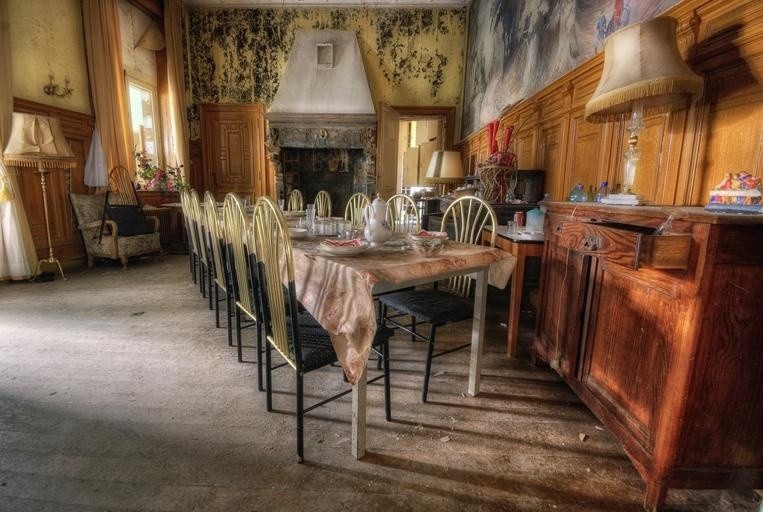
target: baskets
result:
[477,151,518,205]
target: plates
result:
[318,238,370,255]
[407,230,448,242]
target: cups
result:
[277,199,284,212]
[507,220,516,236]
[243,193,255,213]
[297,203,359,241]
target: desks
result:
[480,215,546,369]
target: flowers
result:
[133,148,172,189]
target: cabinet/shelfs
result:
[504,200,763,512]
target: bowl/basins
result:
[288,227,308,239]
[409,239,447,257]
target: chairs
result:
[178,186,228,313]
[222,191,306,394]
[201,189,257,350]
[375,195,499,404]
[67,182,168,267]
[252,194,396,465]
[282,187,425,342]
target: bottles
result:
[592,181,611,203]
[567,183,588,202]
[538,193,554,212]
[524,207,546,234]
[650,211,676,236]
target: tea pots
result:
[362,193,392,243]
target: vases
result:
[133,184,165,211]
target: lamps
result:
[580,14,709,207]
[423,145,465,199]
[2,105,78,279]
